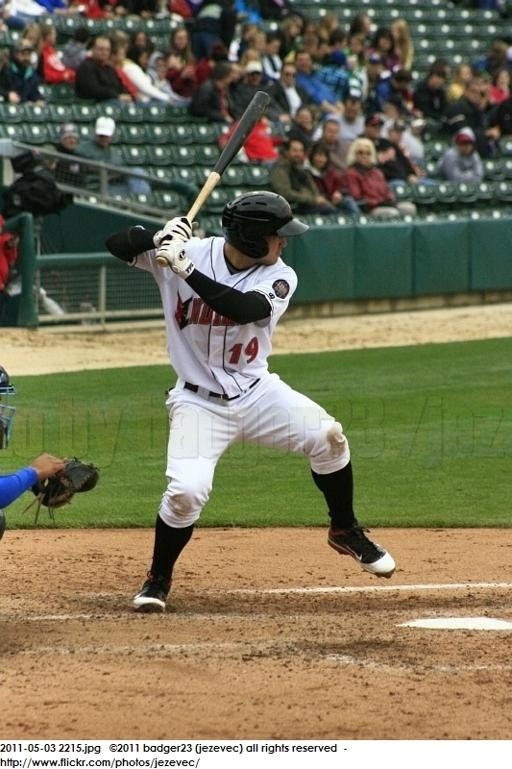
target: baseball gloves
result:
[31,455,98,508]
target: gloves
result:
[154,216,199,280]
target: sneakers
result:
[133,574,172,612]
[326,523,396,578]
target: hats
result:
[456,127,475,143]
[246,61,262,72]
[94,117,116,137]
[364,117,384,126]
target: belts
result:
[184,378,261,401]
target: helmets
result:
[0,365,17,449]
[221,190,309,259]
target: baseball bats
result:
[153,90,270,267]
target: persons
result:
[1,365,99,539]
[2,0,510,220]
[104,191,395,611]
